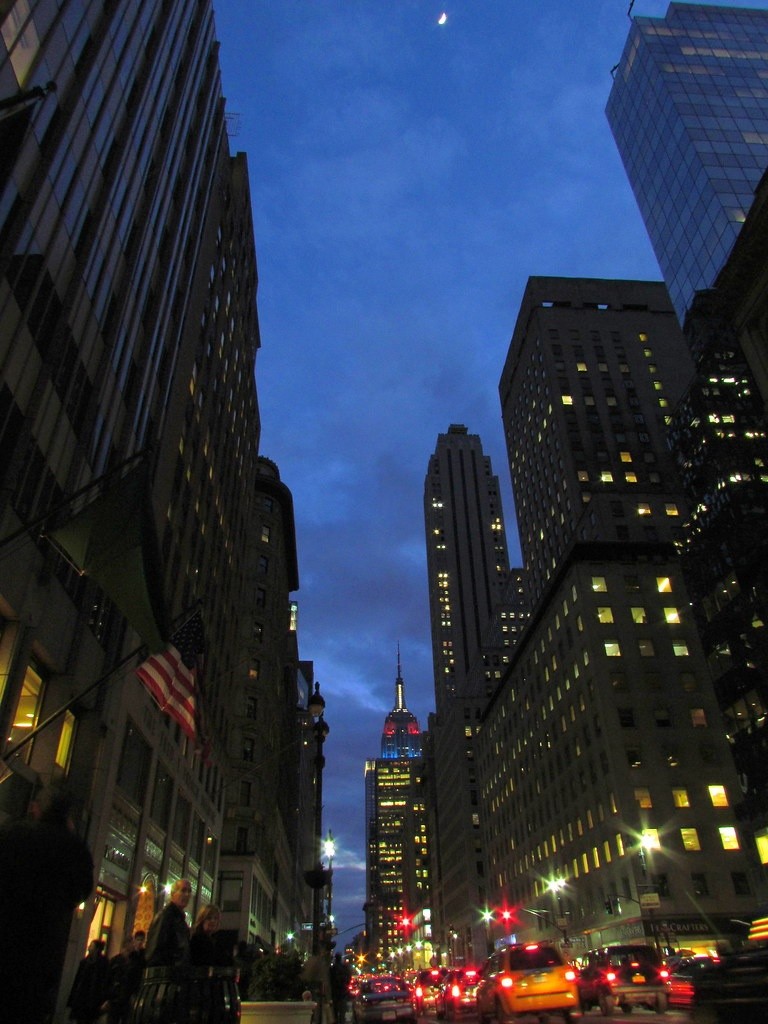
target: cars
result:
[667,957,722,1011]
[352,975,416,1024]
[433,972,486,1023]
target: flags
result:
[135,610,215,769]
[50,465,169,651]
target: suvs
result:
[575,944,674,1017]
[475,940,582,1024]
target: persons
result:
[146,878,191,968]
[190,905,349,1024]
[67,929,147,1024]
[0,793,93,1024]
